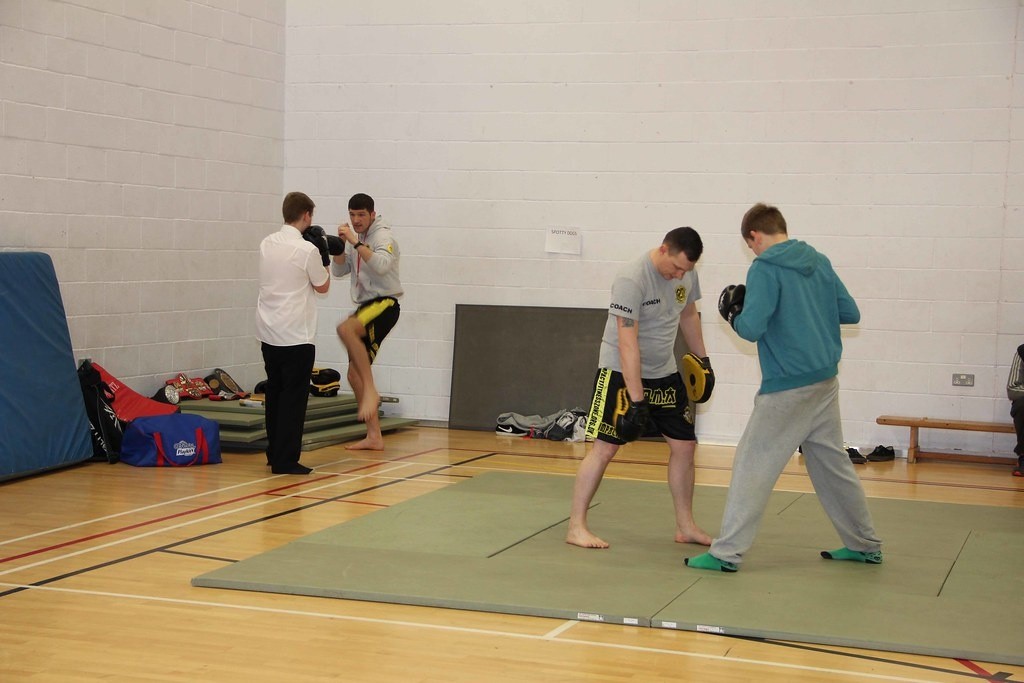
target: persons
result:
[253,190,331,476]
[564,226,719,549]
[331,193,404,449]
[682,201,884,573]
[1006,339,1024,475]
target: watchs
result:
[353,239,365,249]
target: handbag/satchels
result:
[119,413,223,466]
[77,359,124,465]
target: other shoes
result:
[866,445,896,460]
[1012,467,1024,477]
[845,448,867,463]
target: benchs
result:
[876,415,1020,465]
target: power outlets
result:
[952,374,974,387]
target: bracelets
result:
[699,357,712,370]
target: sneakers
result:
[496,424,528,436]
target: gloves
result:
[717,284,745,328]
[302,226,331,266]
[616,397,649,441]
[326,234,345,257]
